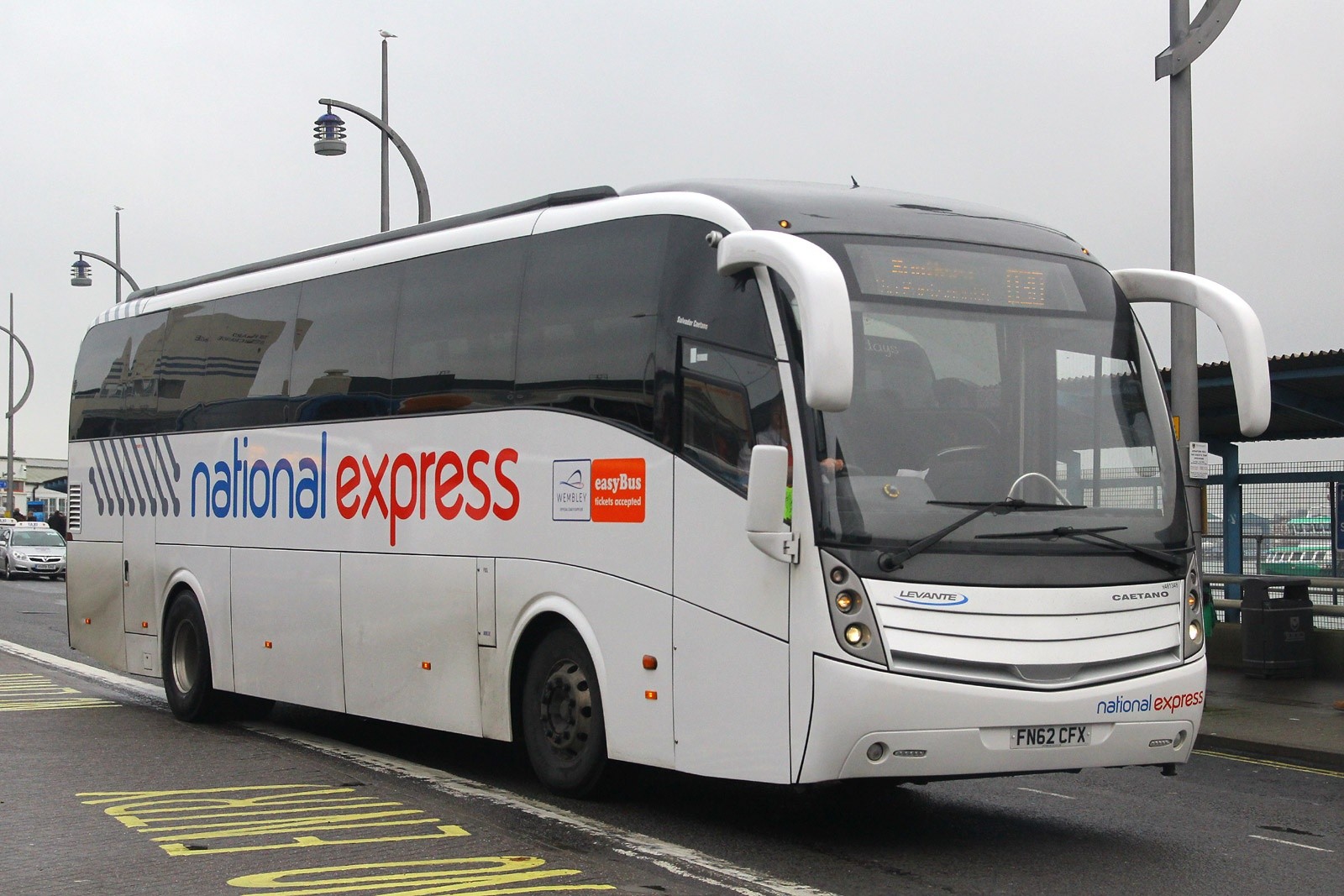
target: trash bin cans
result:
[1240,577,1314,678]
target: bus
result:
[67,182,1272,814]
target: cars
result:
[0,518,66,582]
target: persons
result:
[13,508,66,541]
[735,391,844,486]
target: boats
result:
[1261,507,1333,576]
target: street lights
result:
[71,211,141,304]
[313,38,431,236]
